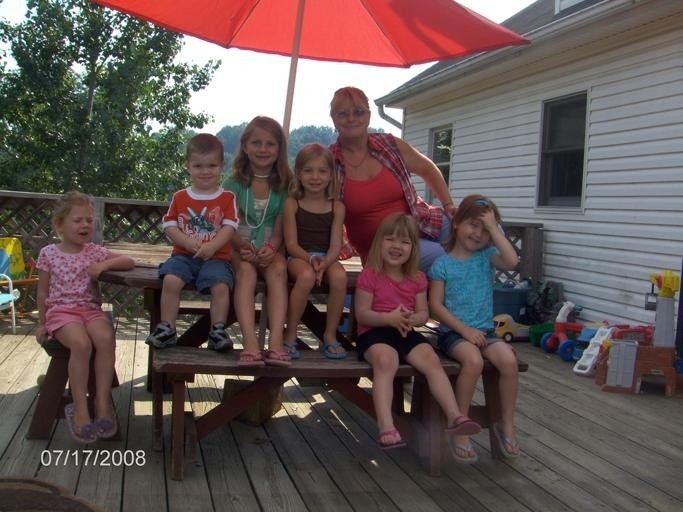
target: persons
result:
[427,194,520,464]
[354,211,481,451]
[146,132,240,351]
[326,87,458,282]
[35,189,136,443]
[222,116,299,368]
[283,142,348,359]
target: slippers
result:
[494,423,521,458]
[94,397,117,438]
[238,351,264,367]
[65,404,97,444]
[265,343,300,365]
[320,342,347,358]
[377,430,406,449]
[445,416,480,463]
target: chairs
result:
[0,236,40,334]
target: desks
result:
[95,242,365,452]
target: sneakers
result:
[208,322,233,351]
[145,322,177,349]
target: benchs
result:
[25,301,528,481]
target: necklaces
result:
[252,171,273,180]
[244,185,274,230]
[340,150,370,174]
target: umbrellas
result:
[91,0,531,352]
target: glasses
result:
[338,108,369,116]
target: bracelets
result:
[441,197,453,209]
[308,253,315,265]
[264,241,277,254]
